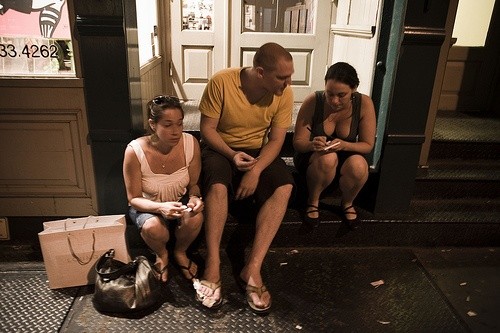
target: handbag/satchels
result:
[94,248,165,319]
[38,213,132,290]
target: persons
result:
[293,62,376,230]
[197,42,296,312]
[123,95,203,284]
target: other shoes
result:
[304,196,321,224]
[340,200,358,224]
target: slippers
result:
[237,268,272,314]
[151,252,169,284]
[194,262,222,311]
[172,251,199,280]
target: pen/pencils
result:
[306,126,329,147]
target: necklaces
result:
[154,139,173,168]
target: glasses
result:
[152,95,179,106]
